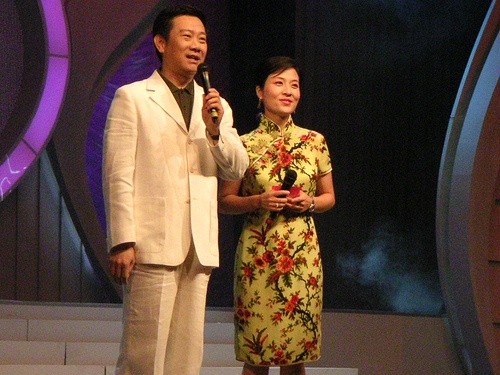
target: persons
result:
[219,55,336,375]
[102,5,250,375]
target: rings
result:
[276,203,279,208]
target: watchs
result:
[306,197,314,214]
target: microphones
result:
[267,170,297,225]
[197,62,219,124]
